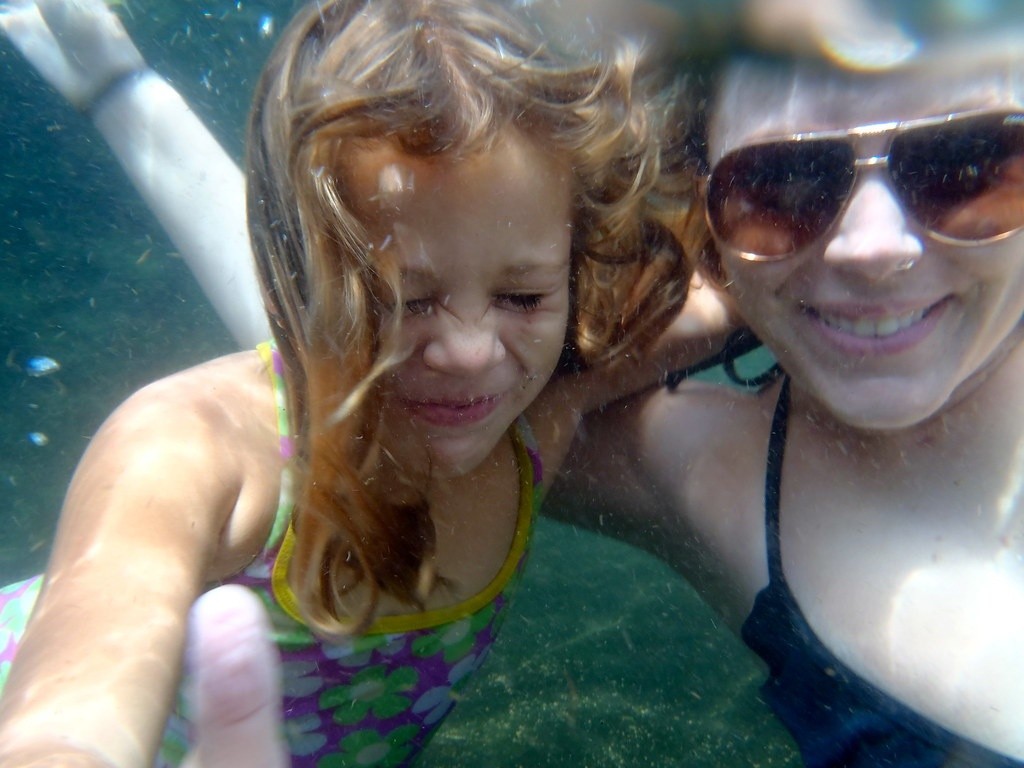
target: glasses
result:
[693,109,1024,262]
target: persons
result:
[0,0,1024,768]
[0,0,752,768]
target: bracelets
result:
[84,66,145,115]
